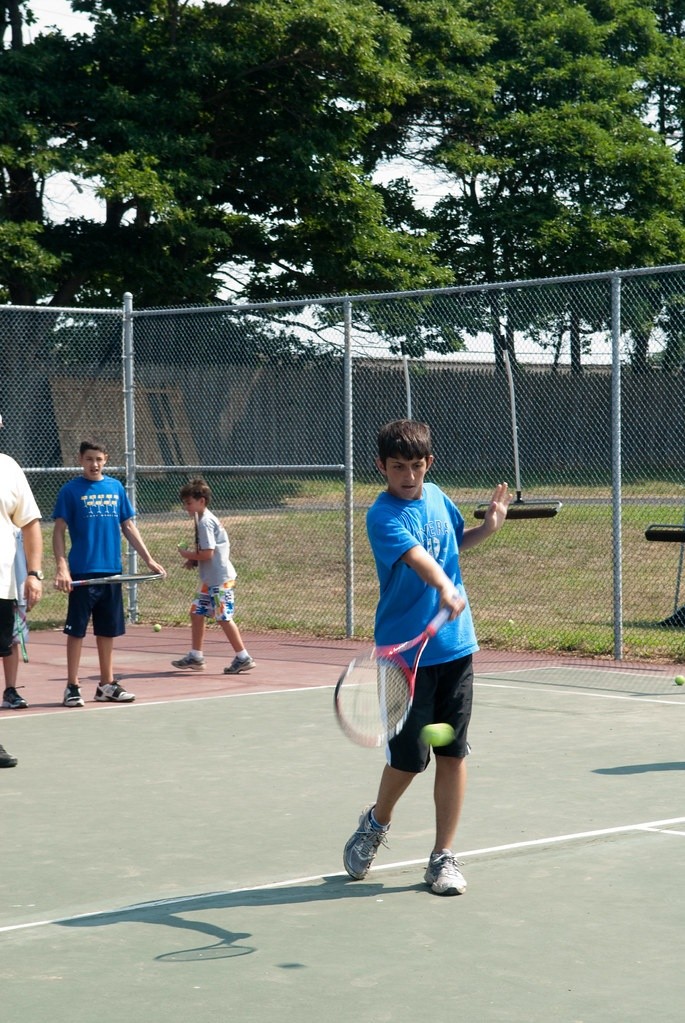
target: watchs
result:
[27,570,44,581]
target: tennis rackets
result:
[194,512,199,567]
[332,586,459,750]
[69,572,163,587]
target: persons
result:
[344,419,512,897]
[54,436,168,706]
[0,413,45,769]
[0,522,30,709]
[172,479,258,674]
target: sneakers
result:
[171,652,206,671]
[224,656,256,675]
[93,680,135,702]
[0,744,19,769]
[423,854,467,896]
[1,687,29,709]
[342,803,391,881]
[63,683,84,707]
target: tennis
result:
[178,543,188,551]
[674,675,685,685]
[153,623,161,632]
[420,723,455,746]
